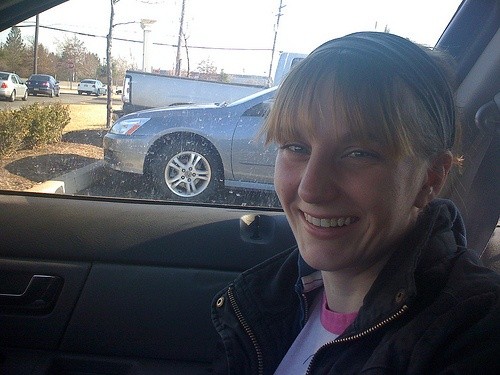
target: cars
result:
[25,74,61,97]
[0,72,29,102]
[102,86,284,204]
[78,79,107,96]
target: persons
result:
[210,30,500,375]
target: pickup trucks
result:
[121,51,315,124]
